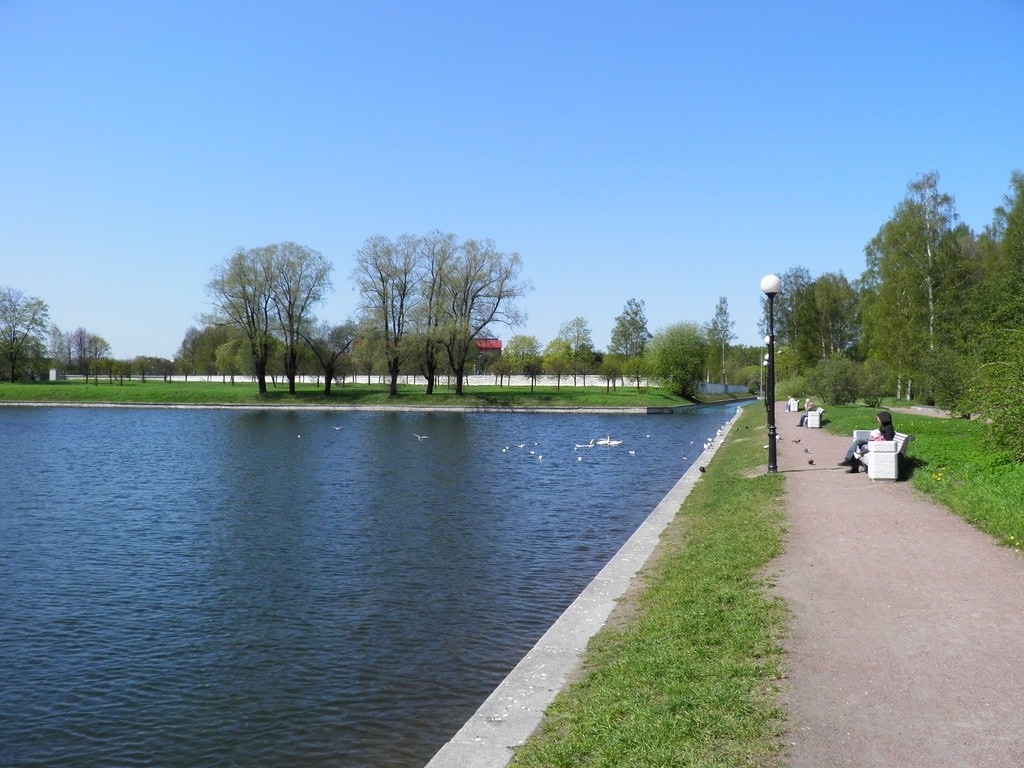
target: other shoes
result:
[837,461,851,466]
[856,446,861,453]
[854,452,860,459]
[845,467,859,473]
[796,425,803,427]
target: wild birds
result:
[776,435,784,441]
[791,439,802,443]
[803,448,811,453]
[628,449,636,455]
[518,442,544,460]
[808,459,814,465]
[746,425,748,429]
[682,420,739,472]
[764,443,771,450]
[412,433,430,441]
[574,433,623,451]
[332,426,342,431]
[577,456,582,461]
[502,446,510,453]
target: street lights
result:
[760,275,785,474]
[762,361,768,406]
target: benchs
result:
[805,407,825,428]
[790,399,801,412]
[853,430,915,483]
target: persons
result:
[786,395,795,412]
[796,399,816,427]
[837,411,894,473]
